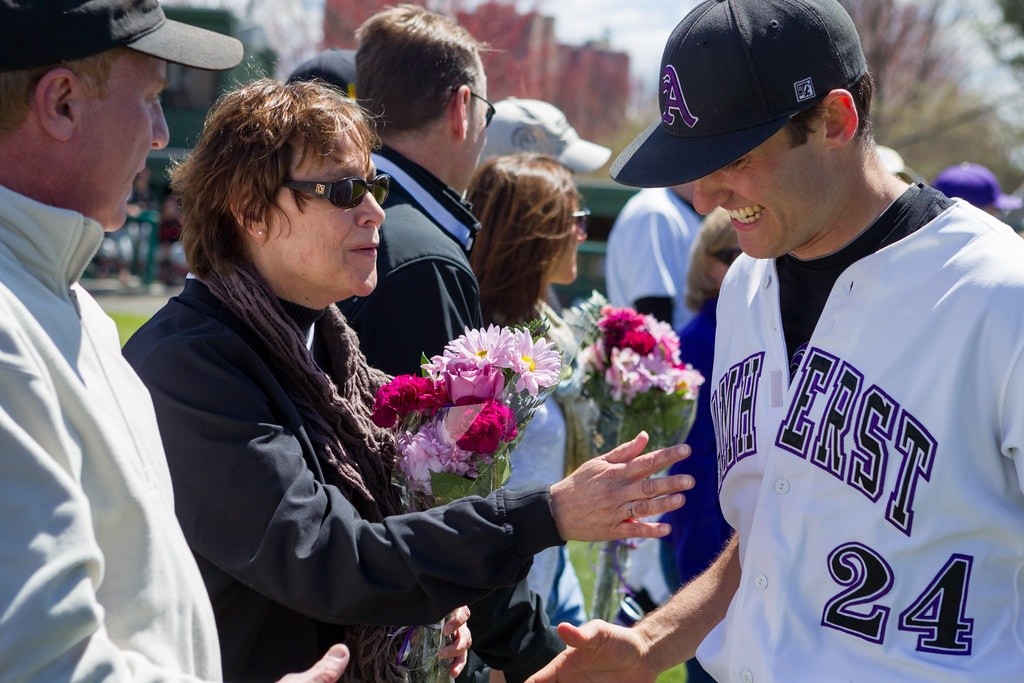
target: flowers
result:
[575,306,707,623]
[372,316,571,683]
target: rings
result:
[627,503,634,518]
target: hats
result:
[7,4,247,75]
[935,159,1024,212]
[285,48,360,103]
[604,1,871,192]
[489,97,612,177]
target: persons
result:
[0,0,1024,683]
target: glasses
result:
[452,87,502,125]
[557,203,595,228]
[266,167,395,211]
[705,248,744,266]
